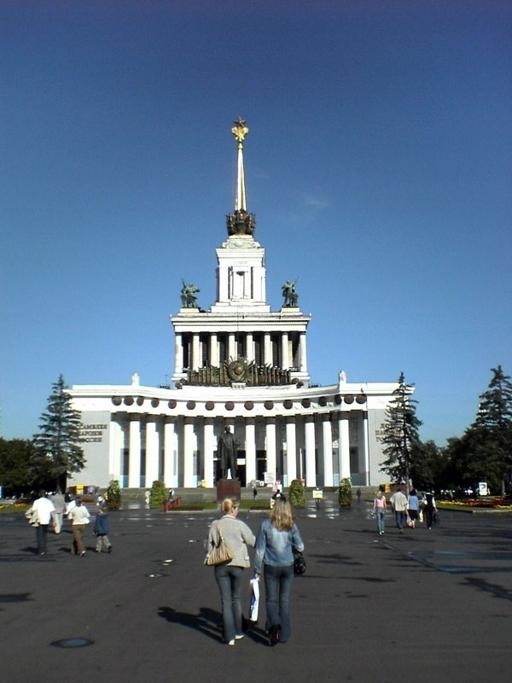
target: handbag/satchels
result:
[248,573,260,624]
[418,509,424,523]
[432,512,440,525]
[406,514,413,527]
[202,518,234,566]
[419,494,428,509]
[291,534,307,576]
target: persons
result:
[167,488,176,500]
[389,486,409,534]
[407,488,420,528]
[144,488,151,510]
[251,495,305,645]
[27,489,91,558]
[205,495,257,646]
[280,278,298,307]
[180,279,200,307]
[93,495,112,553]
[369,490,388,537]
[215,423,241,479]
[420,488,438,531]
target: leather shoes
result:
[270,624,282,646]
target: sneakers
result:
[227,634,244,646]
